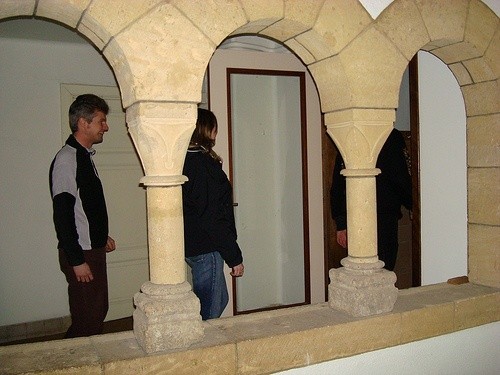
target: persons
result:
[331,106,415,271]
[47,93,117,340]
[180,104,246,323]
